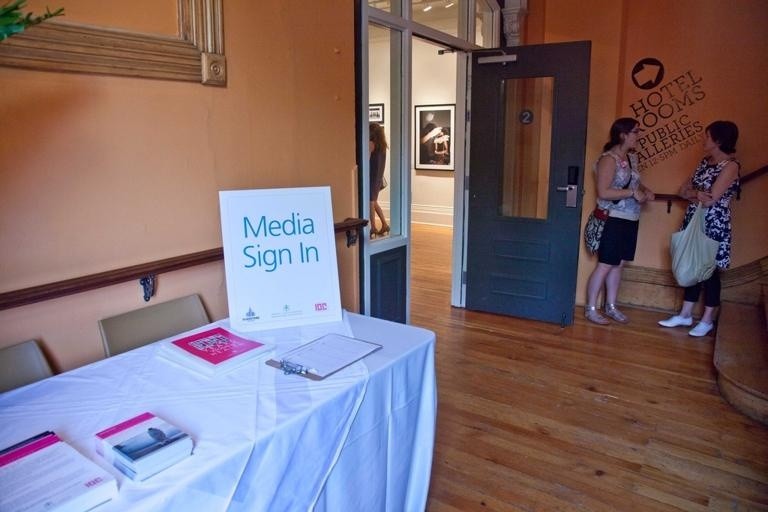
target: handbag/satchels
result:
[583,207,610,256]
[380,176,389,190]
[669,201,720,286]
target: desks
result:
[0,309,437,512]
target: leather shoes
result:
[656,314,695,328]
[688,320,715,337]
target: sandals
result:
[583,303,612,326]
[604,302,630,324]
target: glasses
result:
[628,128,640,135]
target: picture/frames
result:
[0,0,227,88]
[414,105,455,171]
[368,103,384,123]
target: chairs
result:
[0,339,56,395]
[98,294,211,359]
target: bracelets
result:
[695,189,699,197]
[631,188,634,197]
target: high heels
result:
[369,228,379,237]
[377,224,390,236]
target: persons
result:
[368,122,390,239]
[584,117,655,326]
[658,120,741,336]
